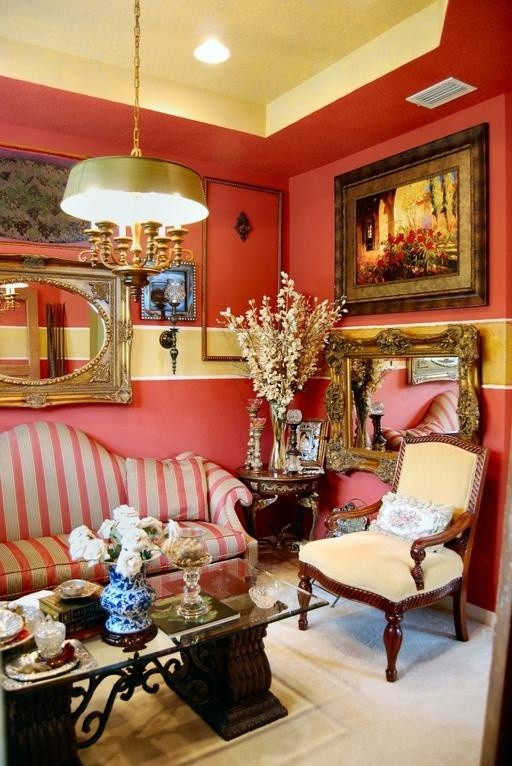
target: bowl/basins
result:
[61,579,86,596]
[1,610,24,643]
[249,583,281,610]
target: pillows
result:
[126,456,211,523]
[366,490,454,556]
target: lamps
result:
[159,281,181,375]
[286,409,302,475]
[60,1,209,304]
[245,398,267,472]
[369,402,387,451]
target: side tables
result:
[235,462,321,555]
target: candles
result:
[4,286,19,294]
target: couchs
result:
[0,421,252,601]
[381,390,458,450]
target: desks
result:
[0,558,329,765]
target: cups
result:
[34,621,66,658]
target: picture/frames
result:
[0,141,122,254]
[287,419,328,468]
[407,358,458,386]
[141,260,197,321]
[333,122,489,313]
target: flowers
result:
[214,270,352,465]
[66,504,183,578]
[350,358,388,433]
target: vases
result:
[354,397,373,451]
[267,401,287,473]
[99,559,157,635]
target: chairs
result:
[299,434,489,680]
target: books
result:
[149,590,241,639]
[39,589,107,643]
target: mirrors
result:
[322,324,481,486]
[0,253,134,408]
[0,288,41,382]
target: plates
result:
[54,580,101,602]
[0,604,43,653]
[5,651,80,682]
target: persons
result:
[303,435,309,449]
[299,430,307,449]
